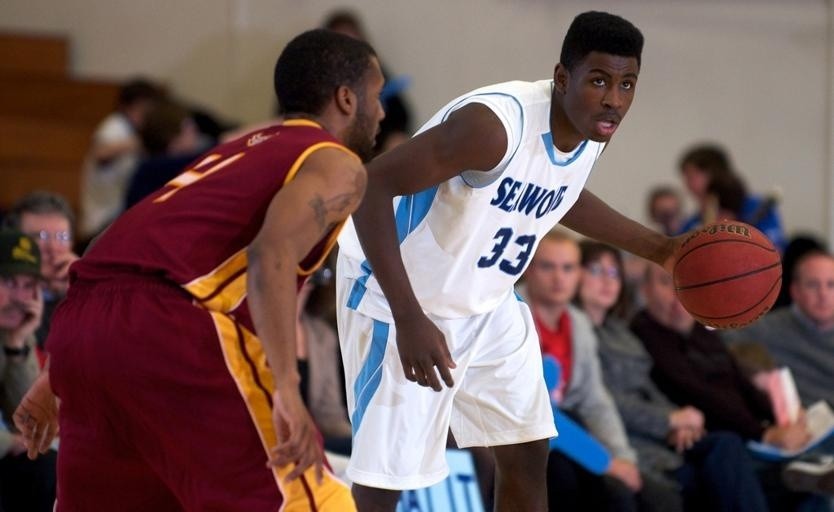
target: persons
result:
[296,69,415,487]
[3,75,235,508]
[514,146,832,508]
[336,10,646,508]
[0,28,385,511]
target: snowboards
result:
[673,219,783,329]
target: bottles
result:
[1,228,47,280]
